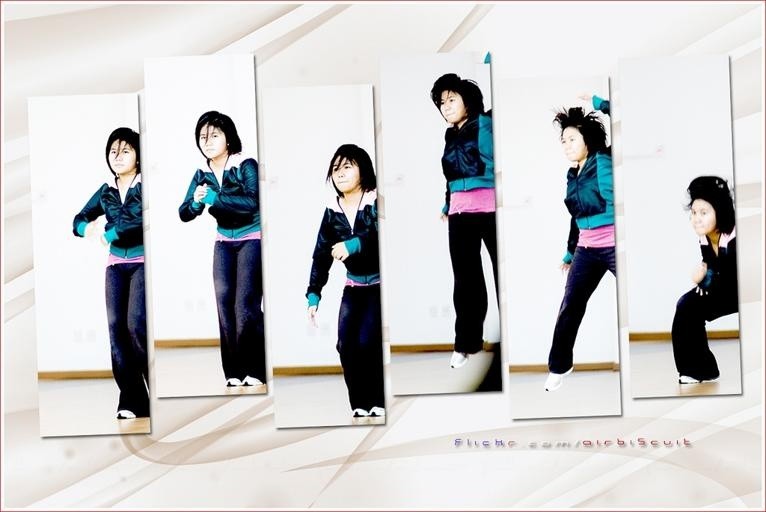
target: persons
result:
[431,51,500,368]
[672,177,740,385]
[178,110,263,386]
[541,94,619,390]
[72,128,148,418]
[304,144,384,417]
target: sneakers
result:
[352,406,385,418]
[225,376,264,387]
[678,374,721,384]
[116,408,138,419]
[544,367,574,392]
[450,348,468,368]
